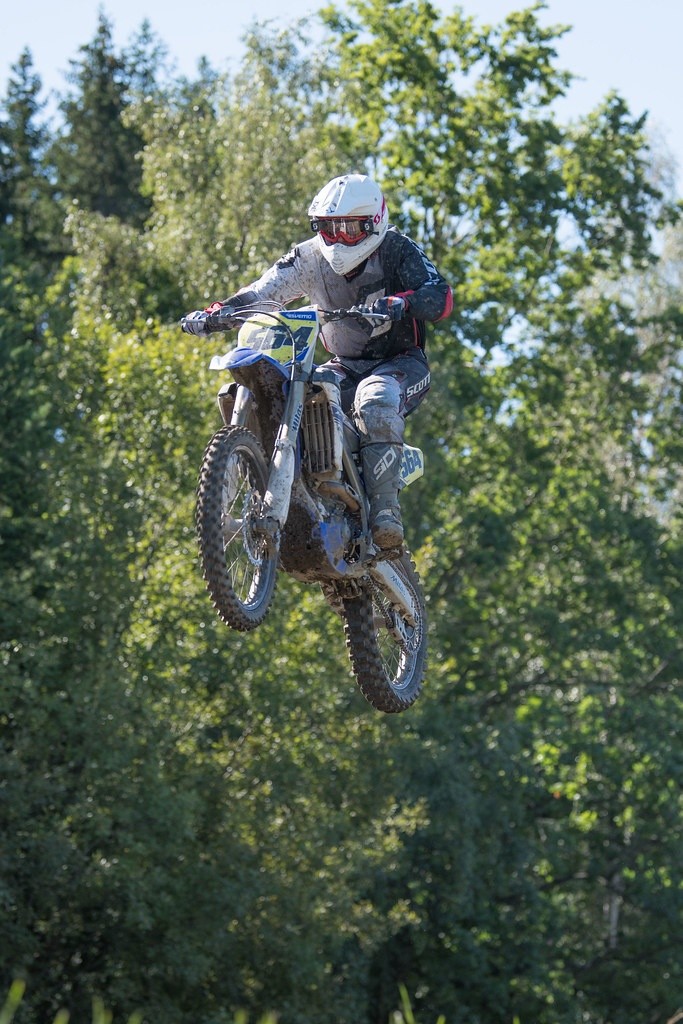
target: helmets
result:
[307,174,389,277]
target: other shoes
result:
[373,509,404,549]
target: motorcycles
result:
[179,309,431,716]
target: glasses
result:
[310,217,374,238]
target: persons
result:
[182,174,452,560]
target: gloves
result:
[182,311,210,336]
[371,296,405,326]
